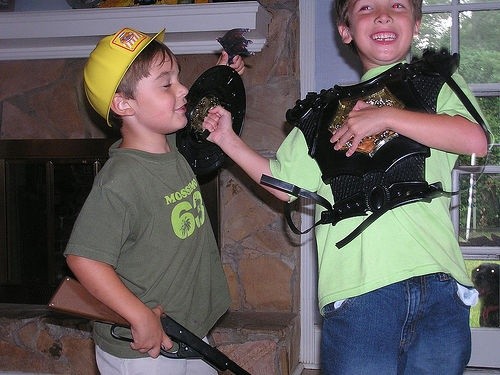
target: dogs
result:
[472,263,500,327]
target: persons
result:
[63,27,248,375]
[202,0,495,375]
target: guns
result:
[49,277,253,375]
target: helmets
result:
[83,27,166,127]
